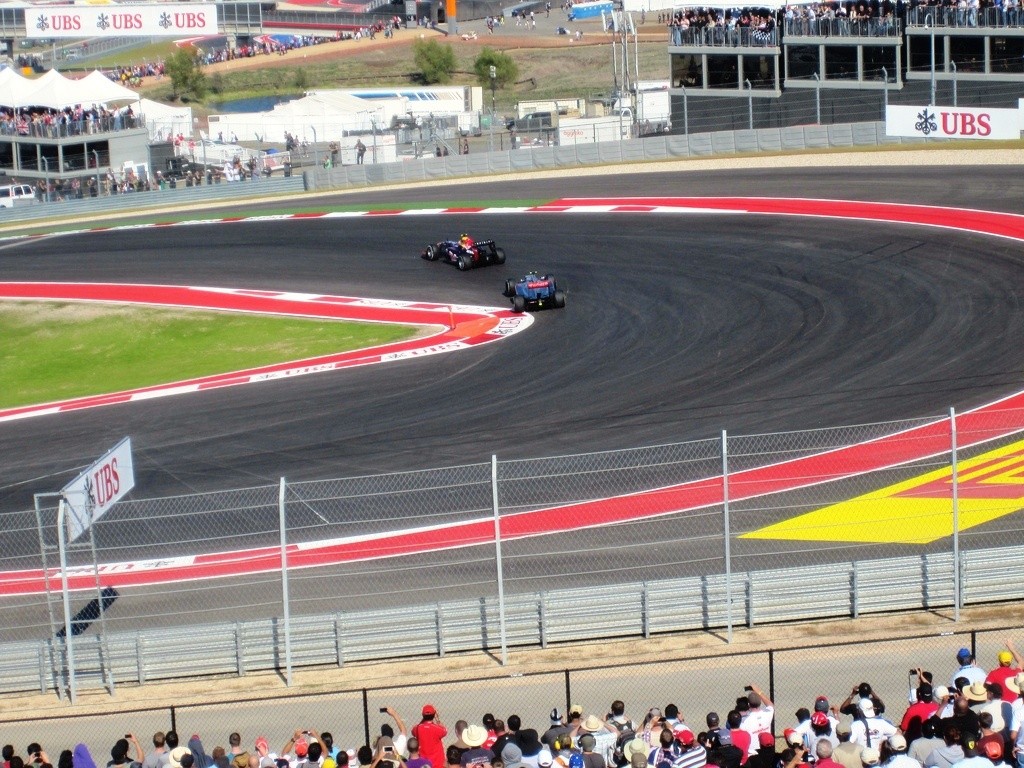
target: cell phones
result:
[744,686,752,691]
[125,734,132,738]
[379,708,387,713]
[658,718,666,722]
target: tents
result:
[0,66,141,123]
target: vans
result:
[0,184,34,208]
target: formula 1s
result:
[506,271,566,312]
[425,239,506,271]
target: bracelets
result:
[291,738,295,742]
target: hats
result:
[932,685,949,704]
[649,708,662,719]
[581,715,604,732]
[322,759,336,768]
[811,713,828,726]
[623,738,650,762]
[707,713,719,723]
[112,739,129,760]
[462,725,488,746]
[956,648,1024,701]
[169,746,191,768]
[295,739,309,756]
[255,737,269,750]
[836,723,851,736]
[672,730,694,745]
[784,729,803,747]
[860,698,875,717]
[962,736,1001,759]
[887,736,907,751]
[758,733,774,746]
[538,750,553,767]
[582,736,595,752]
[814,695,829,712]
[570,705,582,715]
[860,748,879,765]
[422,705,436,715]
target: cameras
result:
[383,746,394,753]
[302,730,310,734]
[910,668,920,675]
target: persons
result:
[644,119,669,133]
[0,104,137,138]
[462,236,469,247]
[323,139,366,169]
[487,1,583,41]
[415,115,423,126]
[510,134,516,150]
[219,131,236,144]
[185,163,273,188]
[423,16,428,28]
[436,138,469,157]
[280,34,330,55]
[37,170,176,203]
[338,16,402,40]
[198,41,277,65]
[659,0,1024,48]
[284,132,293,150]
[107,62,163,85]
[167,133,194,154]
[0,640,1024,768]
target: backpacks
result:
[611,721,635,747]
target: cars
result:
[506,112,557,132]
